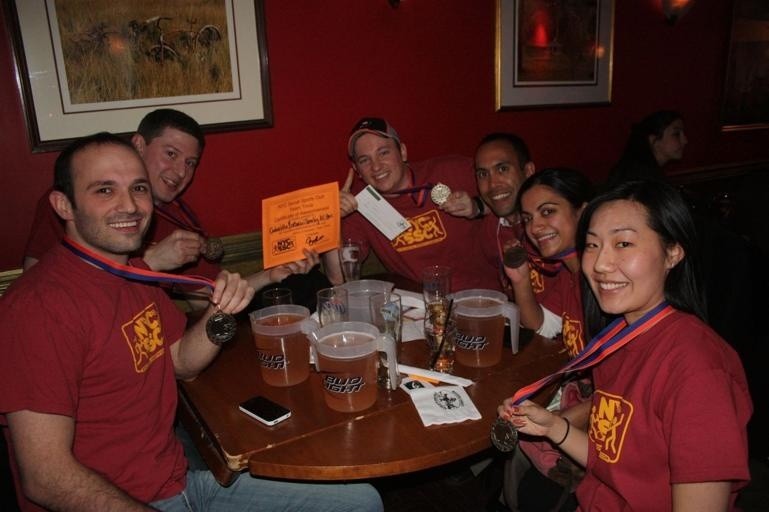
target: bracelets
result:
[473,194,486,217]
[555,417,571,446]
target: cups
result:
[304,321,401,414]
[249,302,320,385]
[260,288,292,306]
[444,287,520,368]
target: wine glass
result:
[318,237,456,379]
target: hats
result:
[346,117,402,163]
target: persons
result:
[23,108,322,313]
[1,130,384,512]
[496,179,754,512]
[498,165,590,336]
[451,131,536,291]
[615,109,689,178]
[321,115,496,295]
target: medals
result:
[489,300,676,453]
[504,245,579,278]
[152,198,224,263]
[405,172,453,211]
[60,236,236,349]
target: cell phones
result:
[238,395,292,426]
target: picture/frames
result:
[3,0,274,156]
[492,1,617,115]
